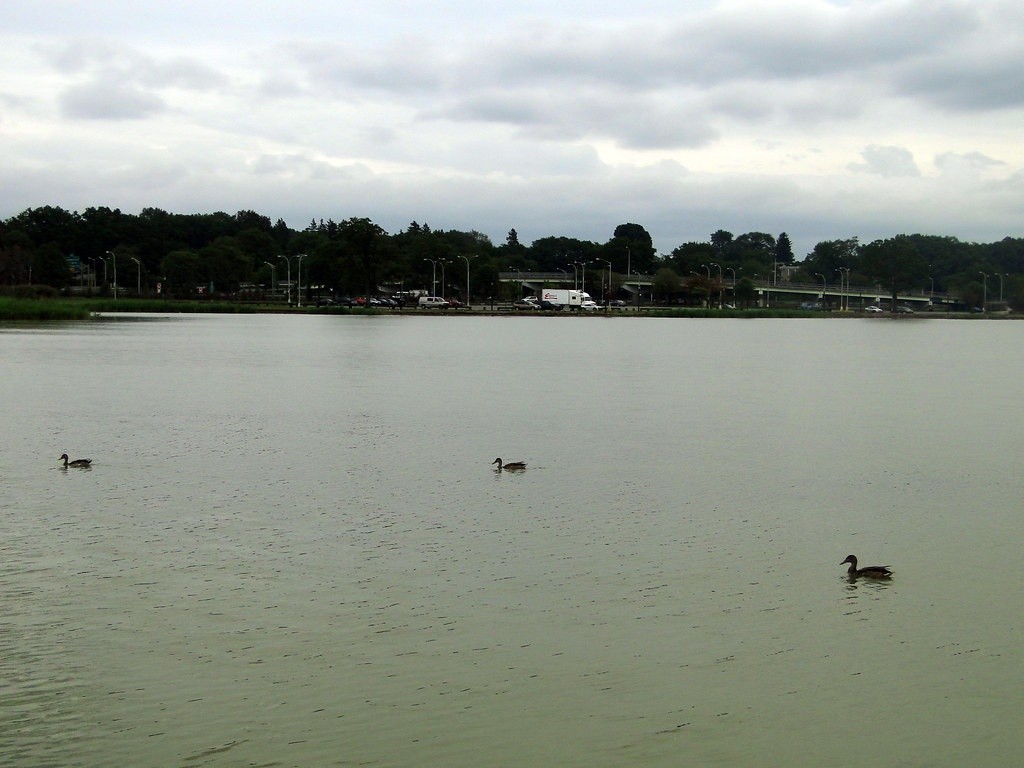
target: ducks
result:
[57,454,92,469]
[839,554,895,581]
[491,458,528,471]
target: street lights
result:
[596,258,611,300]
[509,267,523,301]
[625,246,631,280]
[263,254,308,307]
[815,266,850,313]
[634,271,648,312]
[557,260,593,291]
[978,271,986,303]
[88,251,141,300]
[457,255,479,311]
[424,257,453,299]
[994,272,1003,301]
[701,262,736,310]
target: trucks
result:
[541,289,596,309]
[395,290,429,298]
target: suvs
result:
[521,296,538,302]
[513,300,541,311]
[536,300,562,311]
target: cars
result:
[315,288,406,307]
[897,307,914,314]
[581,303,599,312]
[864,306,883,314]
[611,300,627,307]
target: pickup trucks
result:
[419,297,449,310]
